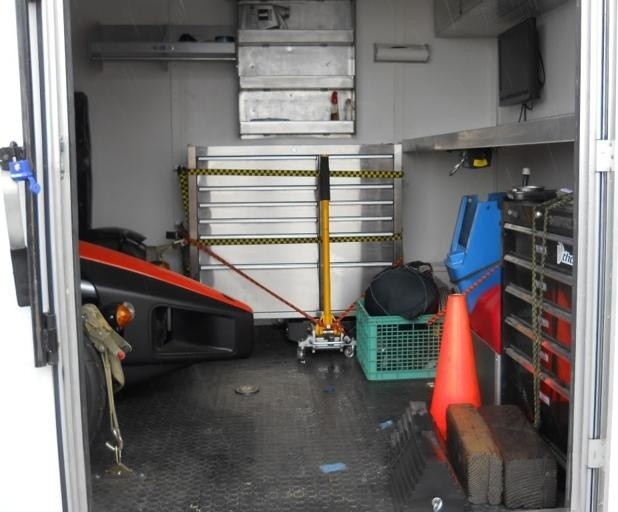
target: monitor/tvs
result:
[497,16,542,109]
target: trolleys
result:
[282,153,358,362]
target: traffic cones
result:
[539,294,573,405]
[432,292,483,443]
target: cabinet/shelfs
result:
[501,200,573,468]
